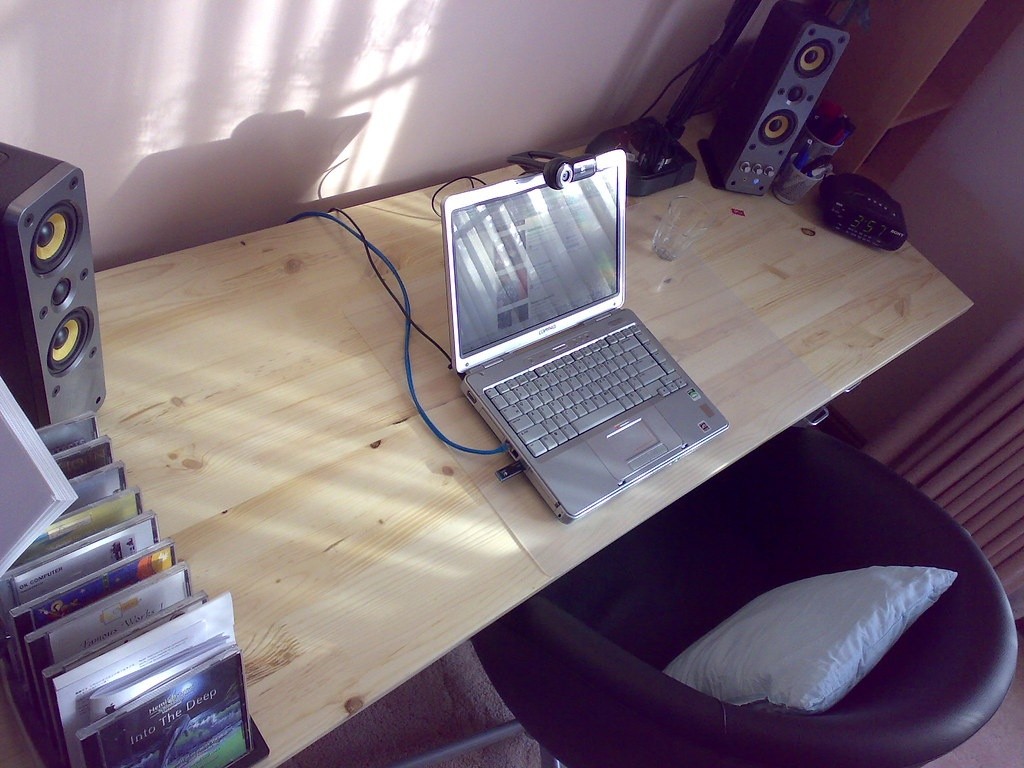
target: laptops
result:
[440,148,731,524]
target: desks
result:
[0,105,976,768]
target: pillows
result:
[661,565,959,715]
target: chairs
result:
[388,427,1017,768]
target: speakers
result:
[0,142,109,430]
[708,0,849,195]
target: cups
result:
[651,196,713,262]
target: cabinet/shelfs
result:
[819,0,1024,195]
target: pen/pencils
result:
[808,112,858,147]
[778,138,832,197]
